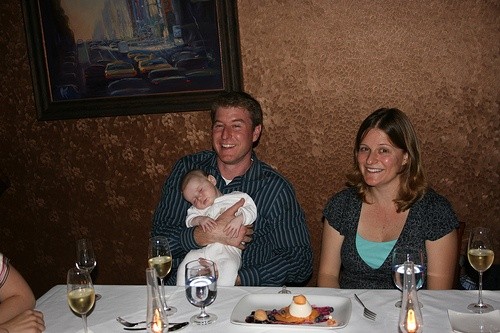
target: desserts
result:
[289,294,312,318]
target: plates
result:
[447,307,500,333]
[230,293,352,330]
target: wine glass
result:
[466,226,496,314]
[66,266,96,333]
[391,247,424,308]
[147,236,177,317]
[185,259,218,326]
[75,238,102,301]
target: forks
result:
[116,315,178,327]
[353,293,378,321]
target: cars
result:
[105,60,137,79]
[163,48,220,89]
[85,64,106,86]
[77,39,120,51]
[128,49,171,75]
[54,50,79,100]
[146,68,191,92]
[107,77,150,95]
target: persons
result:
[144,89,313,286]
[315,107,462,291]
[175,169,258,287]
[0,253,46,333]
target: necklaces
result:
[368,186,404,233]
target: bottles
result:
[146,267,169,333]
[399,261,425,333]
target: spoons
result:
[123,322,189,331]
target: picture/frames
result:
[21,0,244,120]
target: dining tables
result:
[33,284,500,333]
